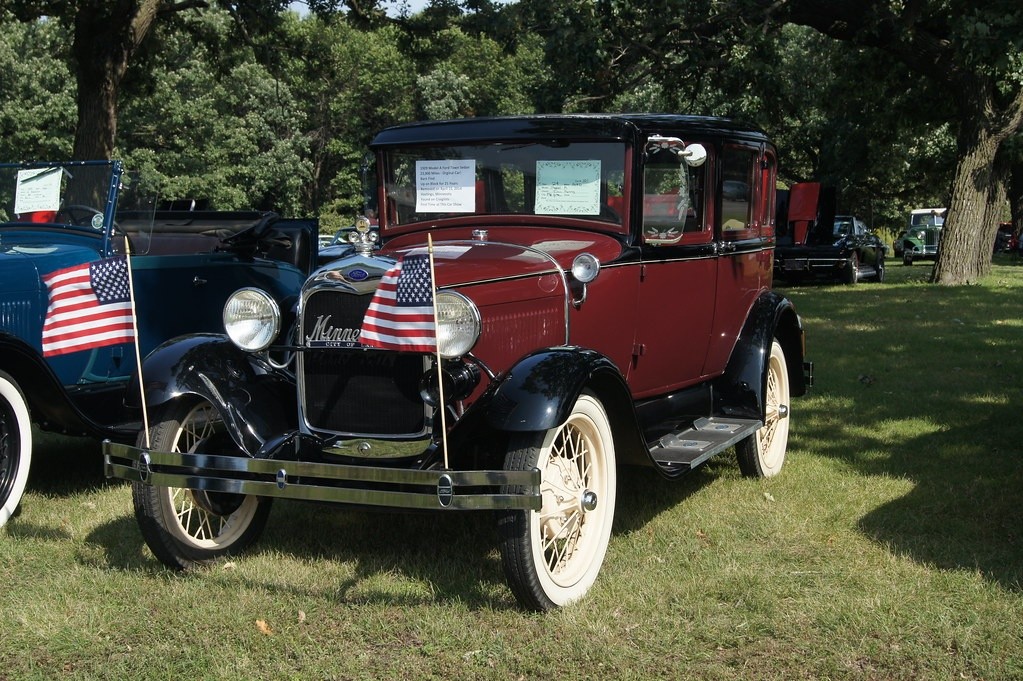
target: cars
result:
[894,204,1013,265]
[101,109,817,615]
[0,160,359,533]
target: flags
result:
[357,253,438,354]
[42,256,138,355]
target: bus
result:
[791,214,886,285]
